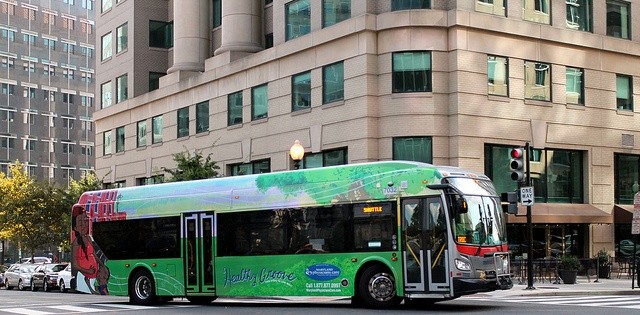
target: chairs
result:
[518,259,529,284]
[544,258,559,283]
[617,259,631,279]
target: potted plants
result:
[555,255,584,284]
[596,248,612,279]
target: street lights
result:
[289,140,305,161]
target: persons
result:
[71,206,101,294]
[97,266,110,295]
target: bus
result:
[71,160,513,309]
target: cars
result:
[31,264,68,292]
[57,264,71,292]
[3,263,35,290]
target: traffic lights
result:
[511,148,529,183]
[500,191,518,215]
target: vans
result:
[19,256,52,265]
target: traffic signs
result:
[630,191,640,233]
[521,186,535,206]
